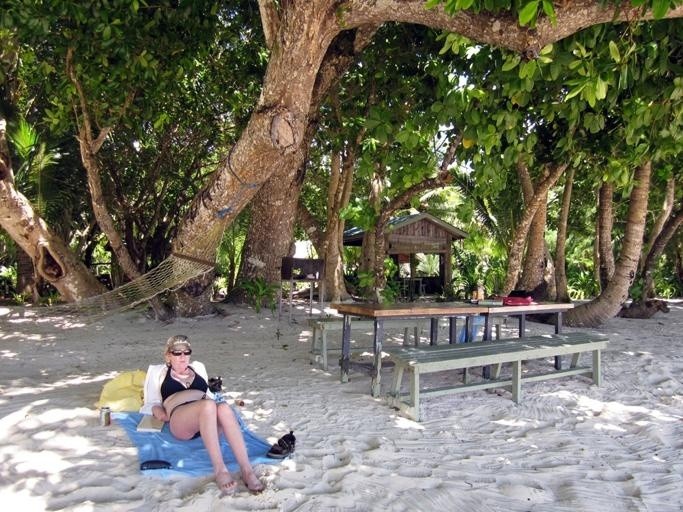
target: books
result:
[135,415,165,433]
[477,299,503,305]
[502,297,529,306]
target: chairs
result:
[397,278,427,297]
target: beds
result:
[0,209,233,324]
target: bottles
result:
[472,286,485,300]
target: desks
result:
[329,299,488,399]
[470,300,576,381]
[278,257,326,324]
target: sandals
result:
[208,377,223,392]
[267,430,296,458]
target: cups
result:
[99,407,112,427]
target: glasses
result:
[168,349,192,356]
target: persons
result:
[142,334,264,492]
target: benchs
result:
[388,331,609,422]
[306,316,509,373]
